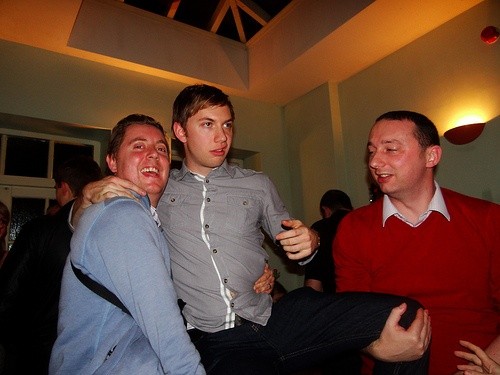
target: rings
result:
[269,284,271,289]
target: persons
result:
[0,157,108,375]
[48,113,209,375]
[271,279,291,305]
[55,84,432,375]
[332,111,500,375]
[304,190,354,292]
